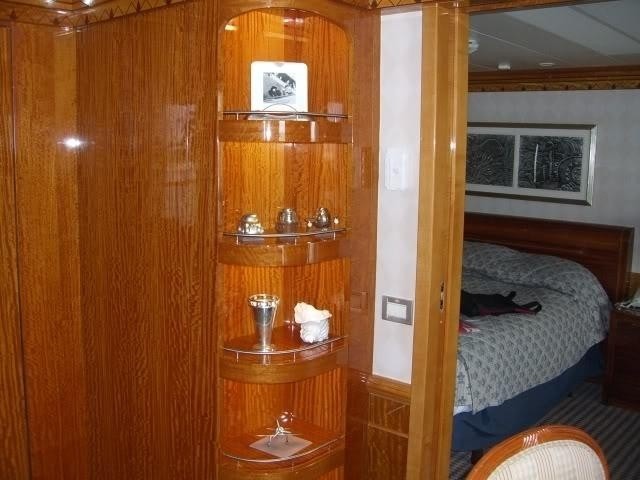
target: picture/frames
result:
[249,61,308,122]
[466,122,597,207]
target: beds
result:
[451,211,635,464]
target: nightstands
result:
[600,300,640,411]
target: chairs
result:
[460,424,611,479]
